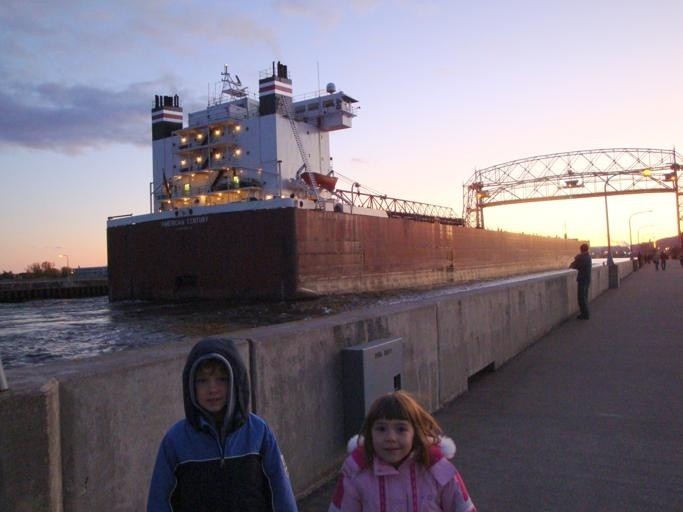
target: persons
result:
[652,247,660,272]
[326,392,476,512]
[569,243,593,320]
[637,251,645,267]
[148,335,300,511]
[660,251,669,270]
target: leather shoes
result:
[577,314,590,321]
